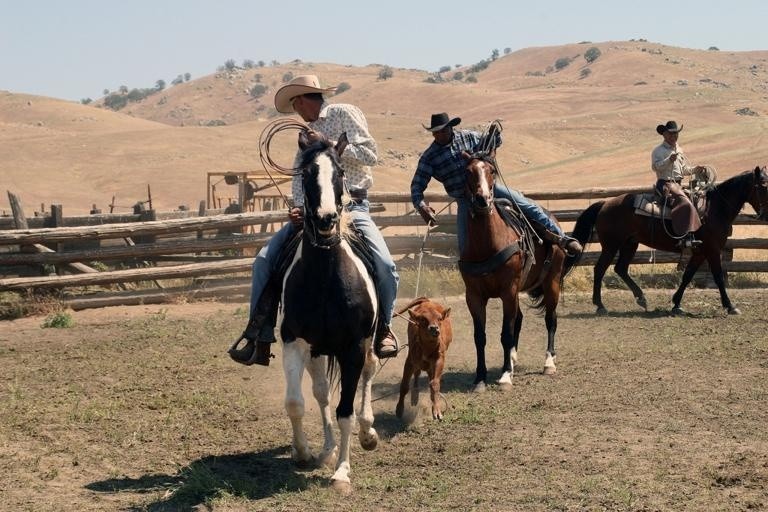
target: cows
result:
[393,296,455,422]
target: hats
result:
[656,121,683,134]
[275,75,338,113]
[421,113,461,132]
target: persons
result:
[651,121,703,247]
[228,74,398,366]
[410,113,583,256]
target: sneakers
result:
[376,329,397,356]
[564,239,583,254]
[229,340,270,365]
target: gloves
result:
[420,206,436,227]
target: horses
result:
[560,165,768,315]
[280,130,379,494]
[458,145,566,395]
